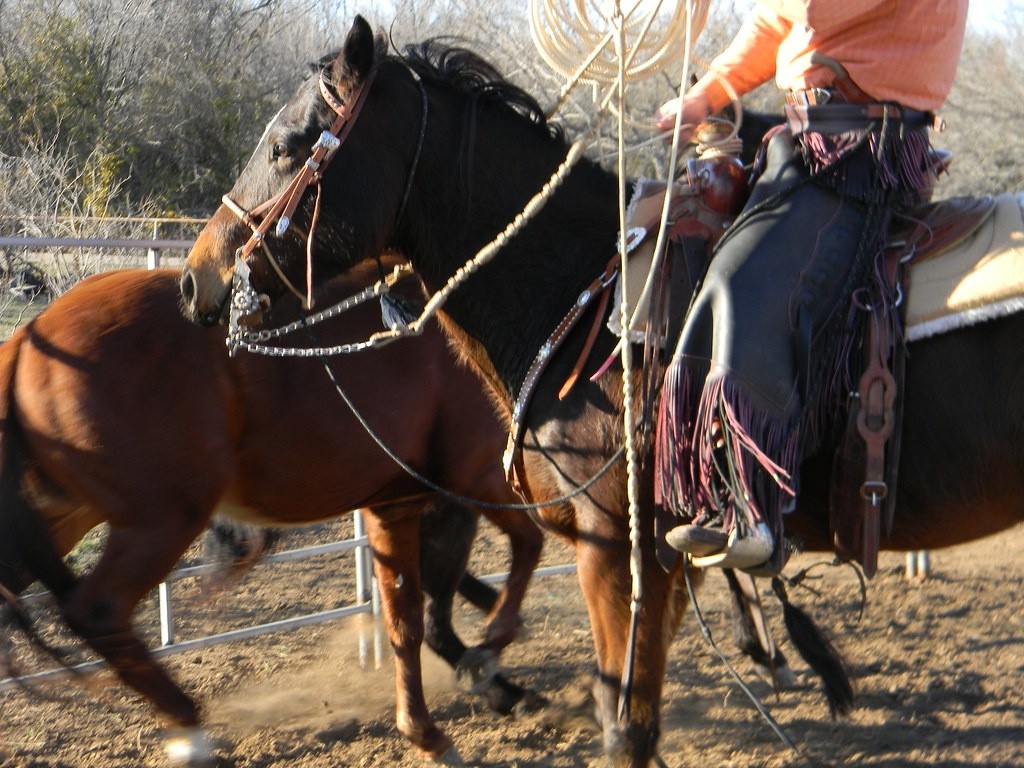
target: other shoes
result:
[665,504,793,578]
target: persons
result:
[653,0,972,577]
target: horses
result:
[174,16,1024,768]
[0,266,544,768]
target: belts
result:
[785,88,847,109]
